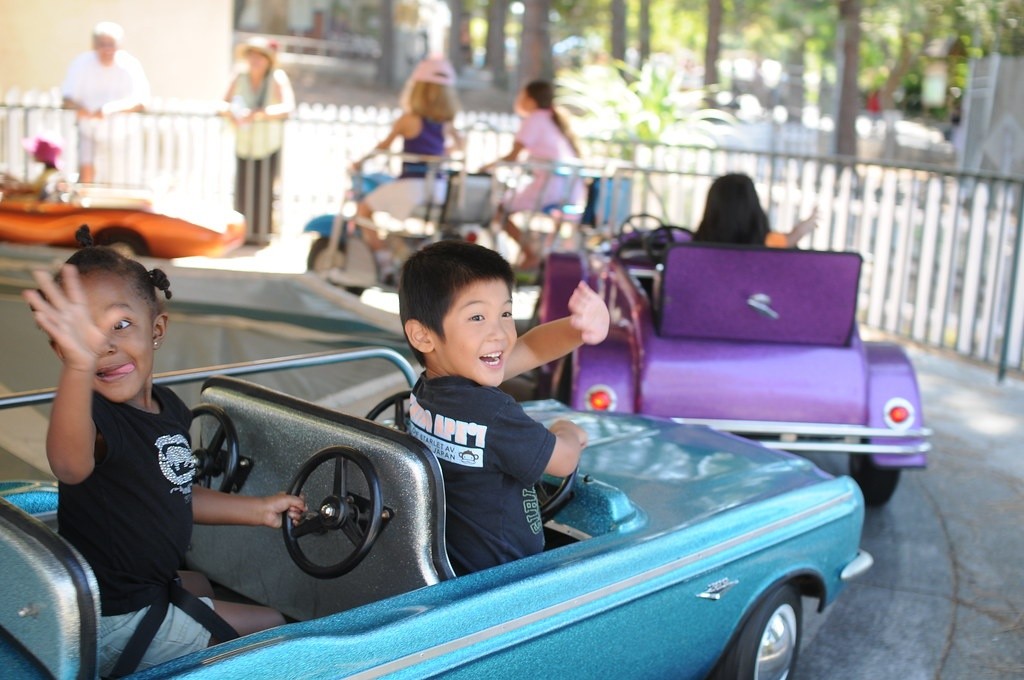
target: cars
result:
[525,215,934,510]
[0,346,876,679]
[2,168,251,270]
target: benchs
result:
[649,244,866,344]
[194,374,462,626]
[0,494,113,680]
[365,171,638,235]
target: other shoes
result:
[379,261,394,284]
[516,255,540,273]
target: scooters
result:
[297,139,612,320]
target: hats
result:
[412,53,456,86]
[235,37,277,67]
[23,134,61,162]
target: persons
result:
[0,134,62,202]
[350,71,456,247]
[690,173,818,250]
[742,58,778,107]
[22,224,308,680]
[58,23,149,183]
[303,10,348,57]
[945,111,961,139]
[479,81,581,272]
[399,240,610,577]
[212,35,296,247]
[868,90,878,111]
